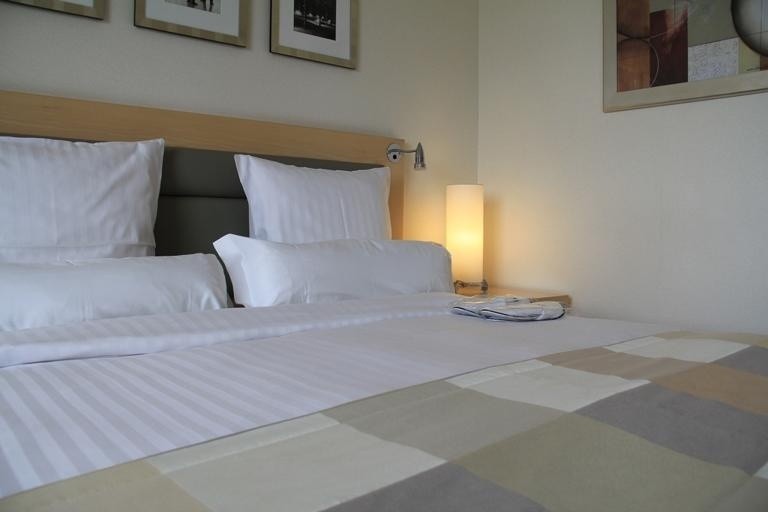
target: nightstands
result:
[456,286,571,309]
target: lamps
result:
[446,184,488,294]
[387,142,426,170]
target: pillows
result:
[0,253,227,332]
[0,137,165,261]
[213,234,455,308]
[233,153,392,244]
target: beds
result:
[1,90,768,512]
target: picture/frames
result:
[270,0,360,69]
[0,0,106,21]
[602,2,768,114]
[134,0,252,48]
